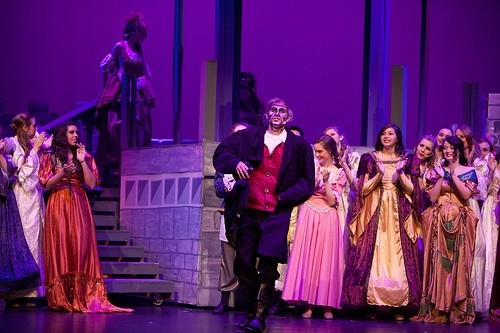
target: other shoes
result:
[369,311,377,321]
[302,312,312,319]
[24,298,38,310]
[324,312,334,319]
[394,314,404,321]
[5,300,20,310]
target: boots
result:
[237,274,260,329]
[243,282,274,333]
[212,290,231,314]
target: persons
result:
[94,13,155,169]
[212,72,500,333]
[0,113,134,312]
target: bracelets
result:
[79,160,85,163]
